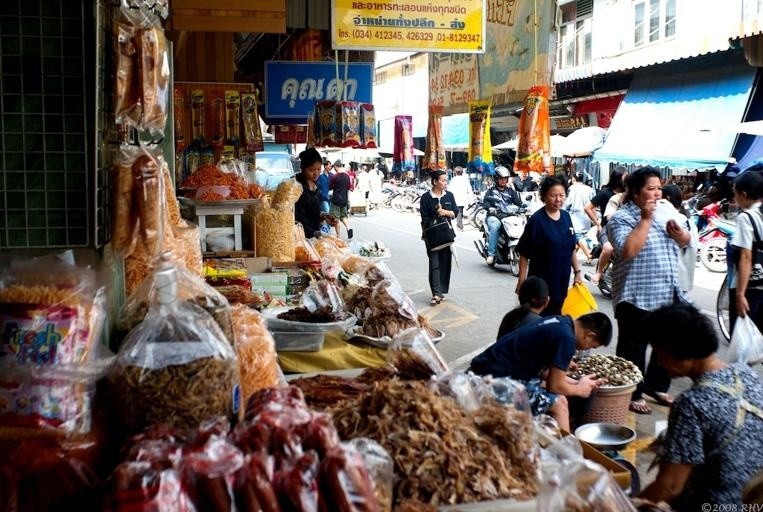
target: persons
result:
[291,148,386,247]
[629,303,763,512]
[464,312,612,433]
[605,167,692,415]
[414,161,763,366]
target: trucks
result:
[253,139,301,196]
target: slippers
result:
[629,397,652,414]
[642,390,674,405]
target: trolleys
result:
[346,188,368,217]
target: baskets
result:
[573,387,637,425]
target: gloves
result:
[488,207,498,215]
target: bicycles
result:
[717,272,731,344]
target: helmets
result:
[493,167,510,184]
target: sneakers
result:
[487,256,494,263]
[348,229,353,239]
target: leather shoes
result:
[429,294,446,304]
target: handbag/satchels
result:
[423,222,456,251]
[727,240,762,287]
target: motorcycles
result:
[584,241,688,301]
[365,178,733,278]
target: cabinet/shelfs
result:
[194,206,244,251]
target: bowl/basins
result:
[398,326,445,349]
[264,307,357,332]
[574,422,637,452]
